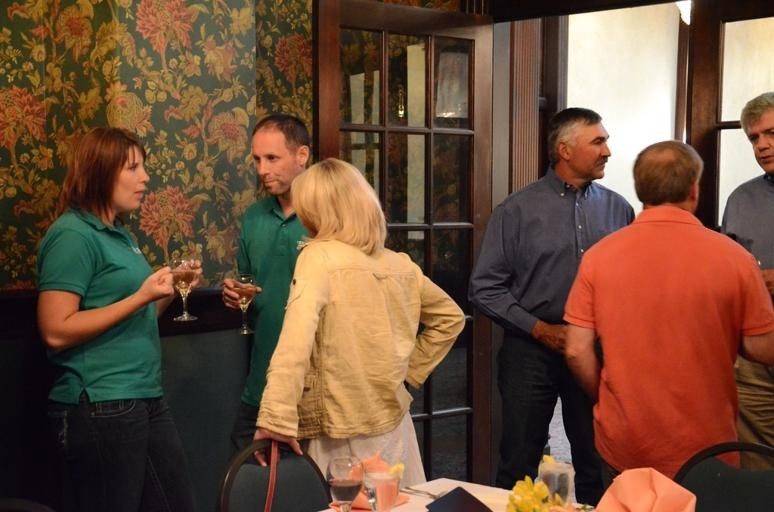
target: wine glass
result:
[233,274,255,335]
[326,456,364,511]
[170,258,199,323]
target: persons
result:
[563,141,774,495]
[720,92,773,471]
[252,158,468,502]
[470,107,634,507]
[35,125,203,512]
[220,114,310,466]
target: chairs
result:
[219,439,333,512]
[673,439,774,512]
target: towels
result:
[595,464,698,512]
[328,453,410,512]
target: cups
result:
[362,471,399,512]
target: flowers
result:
[504,474,592,512]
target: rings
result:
[253,451,258,455]
